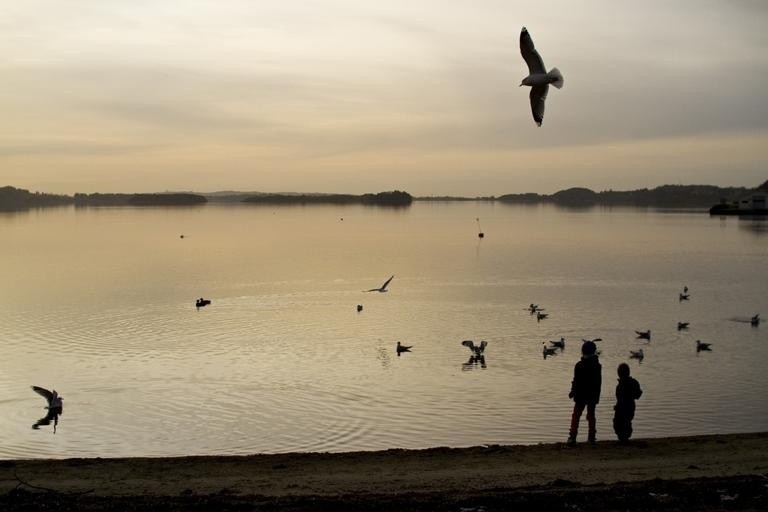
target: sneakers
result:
[566,432,577,445]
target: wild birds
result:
[635,330,650,340]
[678,293,690,298]
[357,304,362,310]
[695,339,712,353]
[676,321,689,330]
[536,311,549,319]
[541,341,555,356]
[360,273,394,296]
[630,349,643,358]
[516,25,563,126]
[31,384,66,411]
[196,297,211,306]
[462,340,488,356]
[584,339,603,344]
[530,304,538,313]
[549,337,565,349]
[397,342,412,351]
[751,313,759,325]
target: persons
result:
[568,339,602,442]
[614,363,642,440]
